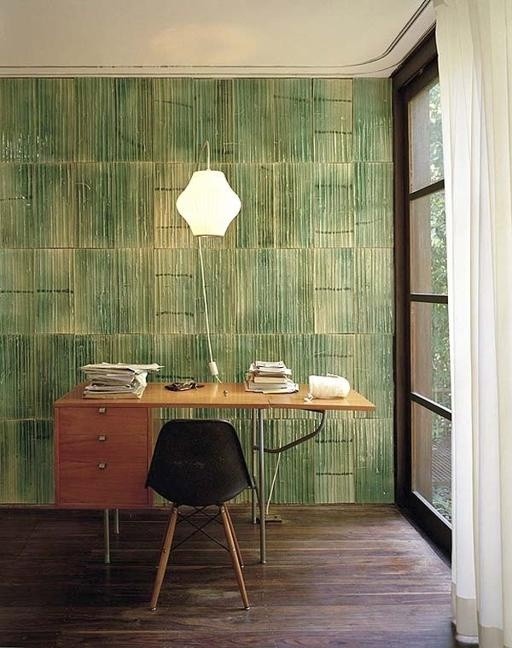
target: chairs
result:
[149,419,250,611]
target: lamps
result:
[176,141,242,382]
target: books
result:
[243,361,299,393]
[80,362,166,399]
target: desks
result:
[52,382,375,564]
